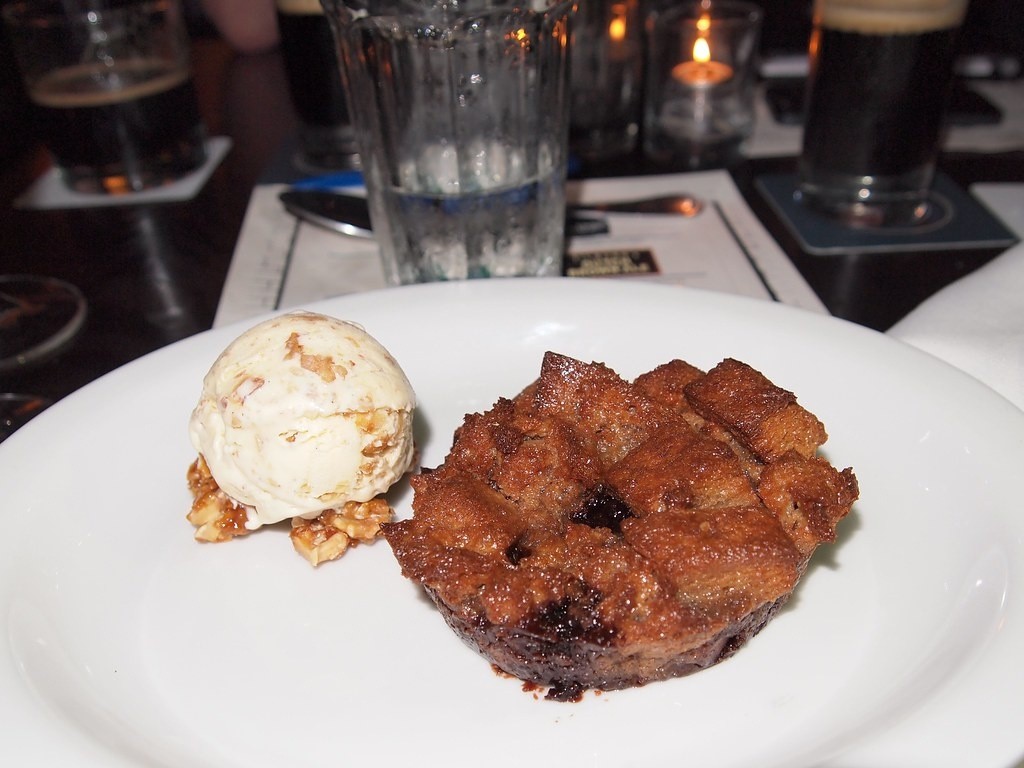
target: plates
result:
[0,278,1024,768]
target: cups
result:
[802,1,966,224]
[1,0,207,194]
[319,0,572,296]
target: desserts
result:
[189,311,421,529]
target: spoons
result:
[286,190,702,231]
